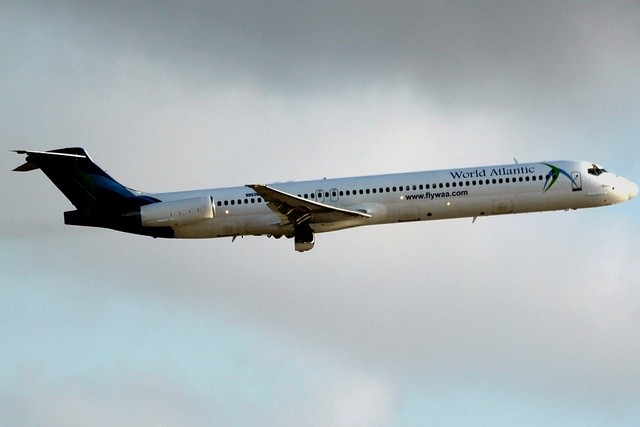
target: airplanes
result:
[9,146,638,252]
